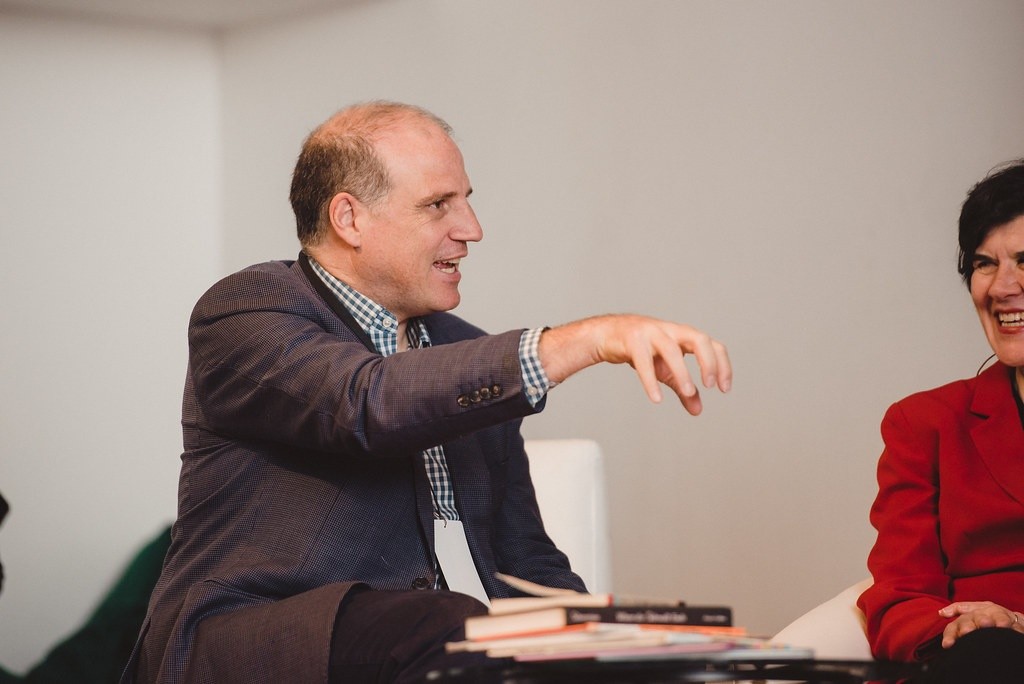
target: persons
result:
[119,98,733,684]
[842,153,1024,684]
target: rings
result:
[1012,613,1019,625]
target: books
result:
[442,570,815,668]
